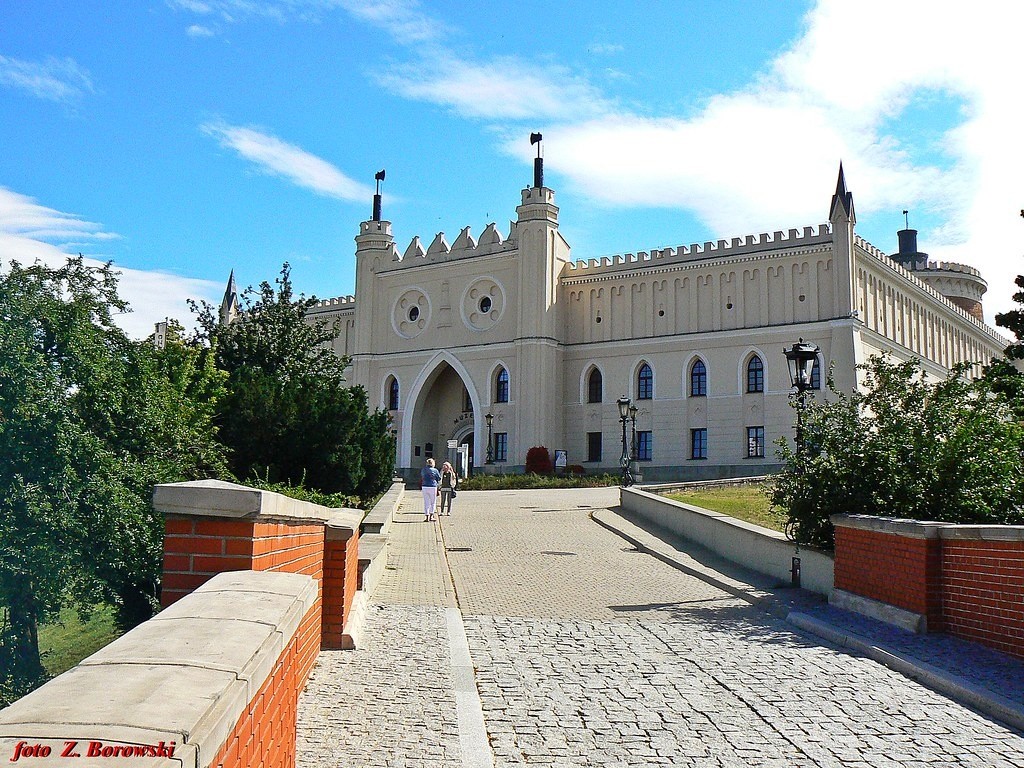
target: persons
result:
[439,461,456,516]
[421,458,441,522]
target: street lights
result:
[485,411,495,464]
[615,395,634,486]
[628,405,639,461]
[780,333,822,472]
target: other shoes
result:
[447,512,450,516]
[438,513,444,516]
[423,519,429,522]
[430,518,436,521]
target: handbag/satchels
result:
[451,487,456,498]
